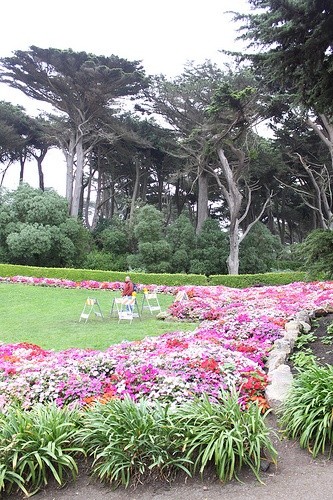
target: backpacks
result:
[133,283,136,292]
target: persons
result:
[121,276,135,312]
[172,289,188,302]
